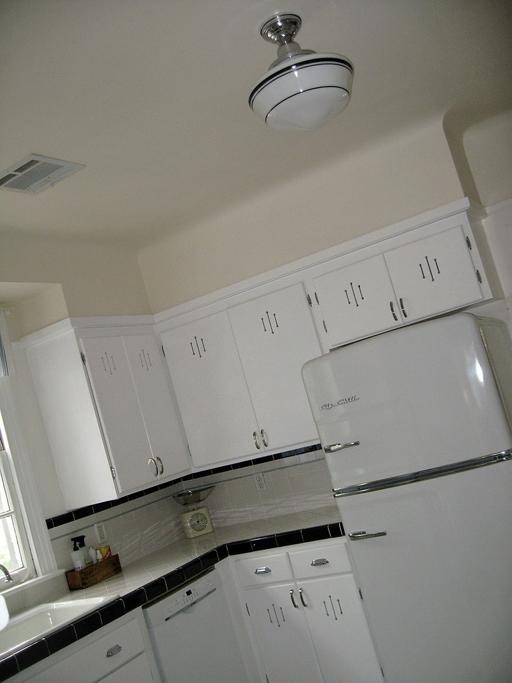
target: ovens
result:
[142,566,248,682]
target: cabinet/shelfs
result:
[148,261,333,476]
[292,194,505,358]
[0,599,165,683]
[13,307,196,515]
[219,515,386,681]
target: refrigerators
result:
[301,312,512,682]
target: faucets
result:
[0,563,15,584]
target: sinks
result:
[0,594,120,660]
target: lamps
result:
[246,6,358,134]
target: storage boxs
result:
[64,552,124,592]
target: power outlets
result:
[251,470,266,490]
[94,520,109,546]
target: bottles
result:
[69,544,103,570]
